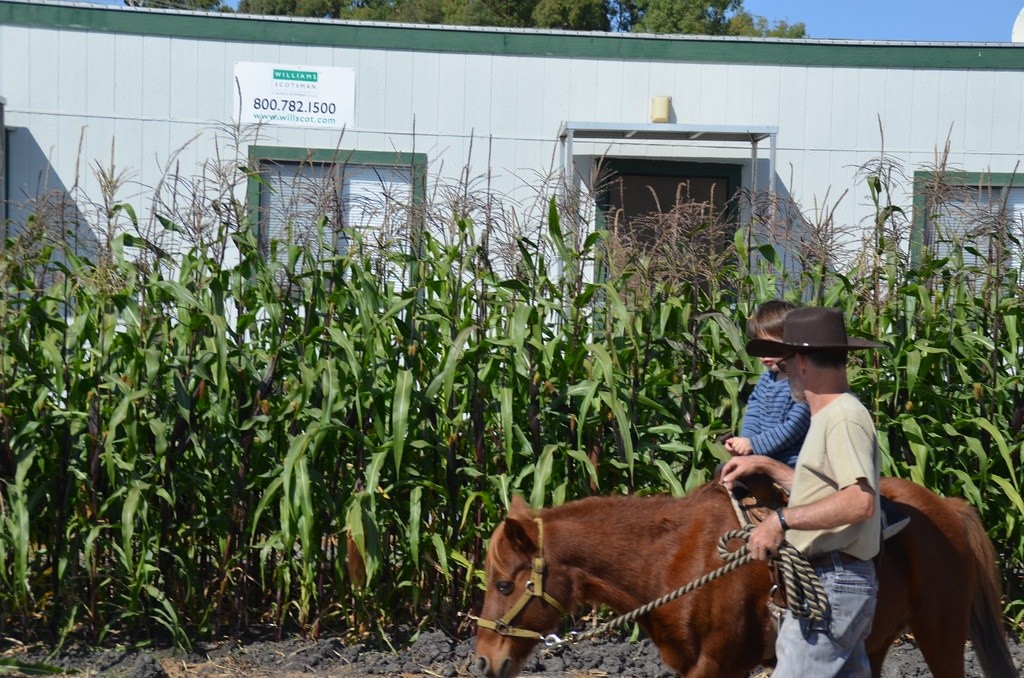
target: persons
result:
[720,305,887,678]
[725,300,811,467]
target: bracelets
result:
[776,508,790,531]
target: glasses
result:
[776,351,812,372]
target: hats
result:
[745,307,887,358]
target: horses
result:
[476,477,1020,678]
